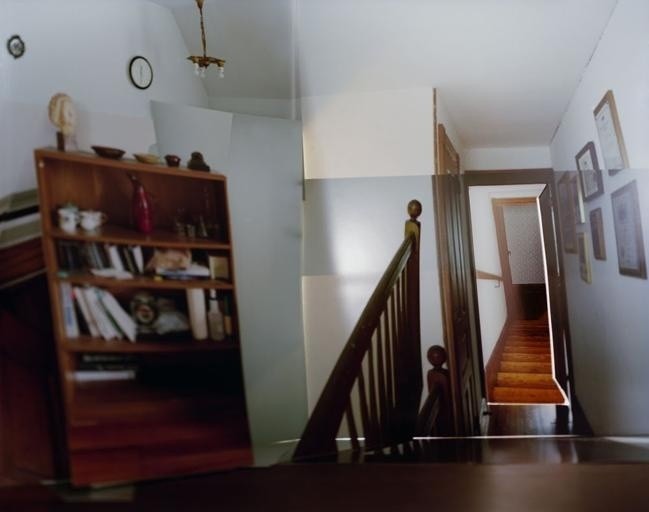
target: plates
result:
[134,152,161,163]
[91,146,126,160]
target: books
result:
[51,235,147,340]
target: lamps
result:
[186,0,226,76]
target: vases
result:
[129,171,156,232]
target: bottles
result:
[206,290,233,344]
[132,182,155,236]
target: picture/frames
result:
[554,89,649,286]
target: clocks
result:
[130,56,154,90]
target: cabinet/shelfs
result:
[24,142,254,490]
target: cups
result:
[57,207,111,234]
[166,155,180,167]
